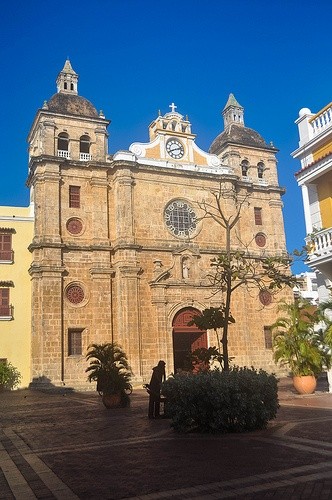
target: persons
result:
[148,360,166,420]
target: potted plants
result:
[84,342,134,409]
[270,295,332,394]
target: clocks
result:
[165,137,186,159]
